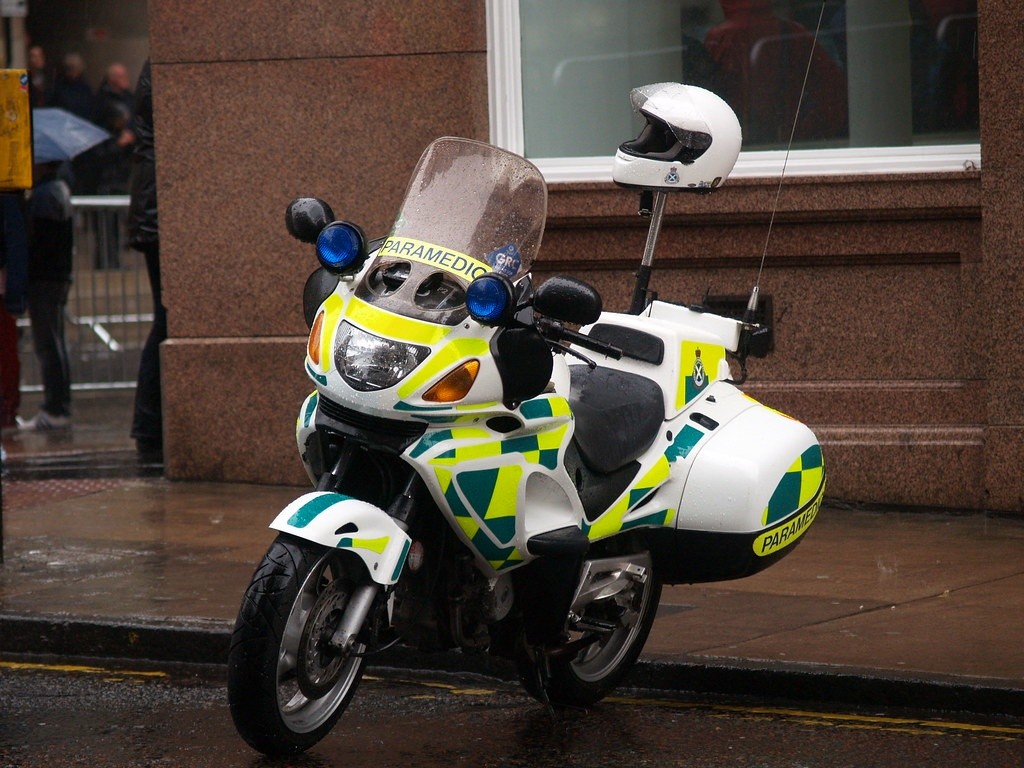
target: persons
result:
[702,1,975,147]
[46,52,93,100]
[125,56,164,470]
[18,159,76,432]
[89,96,133,272]
[91,63,138,214]
[0,192,31,435]
[23,44,52,107]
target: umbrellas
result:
[28,106,113,167]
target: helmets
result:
[612,80,743,193]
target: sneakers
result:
[134,439,164,468]
[17,410,75,433]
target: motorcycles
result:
[222,134,831,764]
